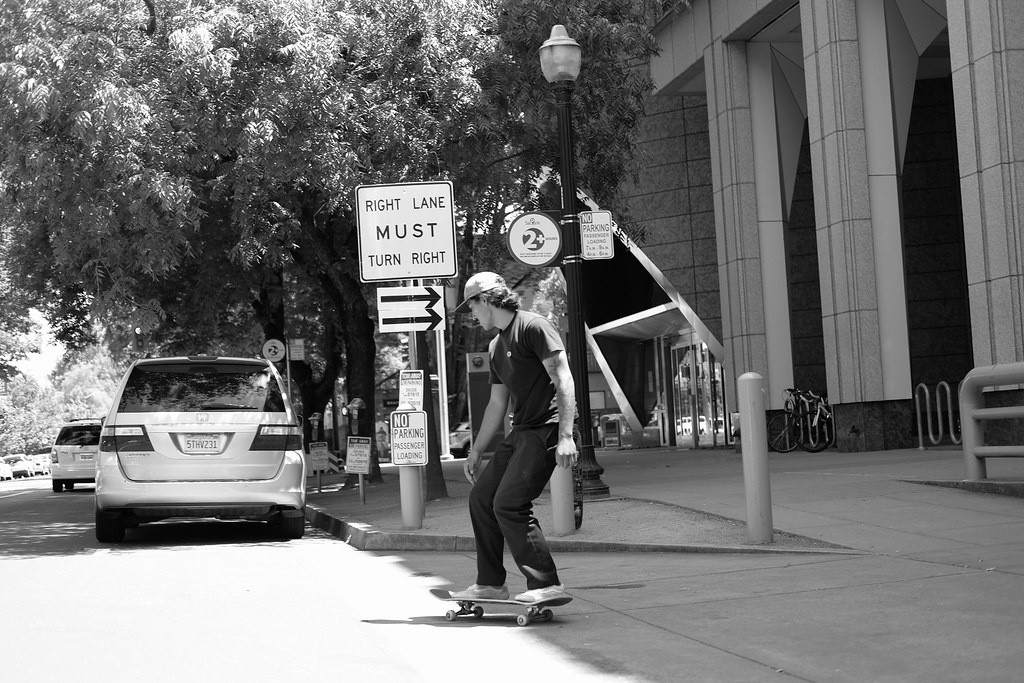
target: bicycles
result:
[767,385,833,454]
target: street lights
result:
[538,24,610,498]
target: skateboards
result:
[429,588,573,626]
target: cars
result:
[674,413,726,437]
[0,452,51,482]
[94,353,308,543]
[49,418,104,492]
[448,420,471,459]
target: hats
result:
[454,272,505,313]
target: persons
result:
[453,271,578,602]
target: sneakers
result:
[453,582,509,600]
[514,582,566,601]
